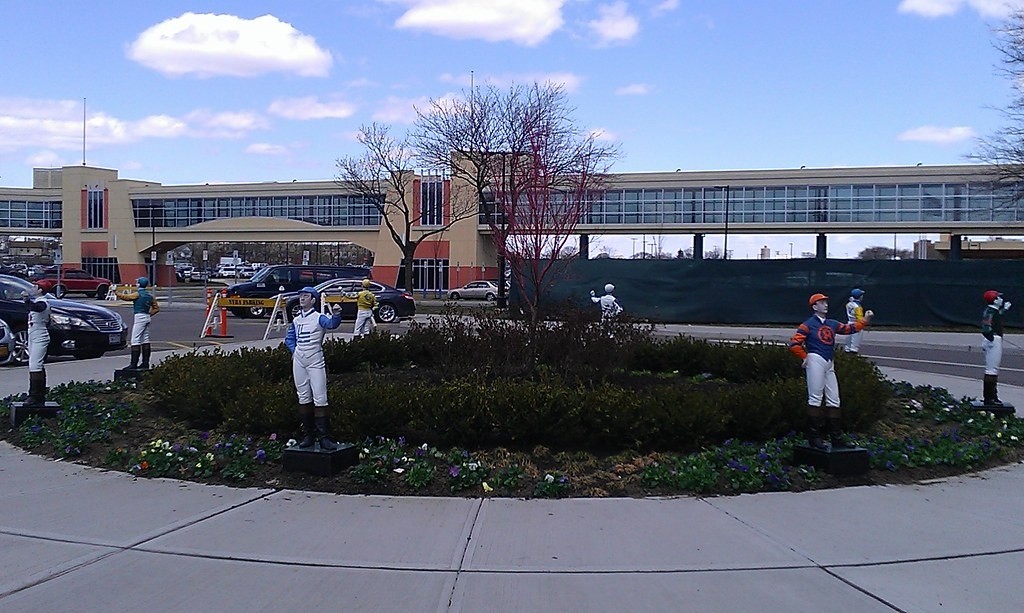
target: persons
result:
[590,283,623,339]
[844,288,865,356]
[109,276,160,371]
[283,286,342,450]
[338,279,379,340]
[789,294,875,450]
[20,279,52,408]
[981,290,1012,407]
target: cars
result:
[265,278,417,324]
[14,263,59,277]
[0,275,128,359]
[0,319,16,367]
[29,268,112,300]
[447,280,509,302]
[217,263,373,319]
[174,260,270,283]
